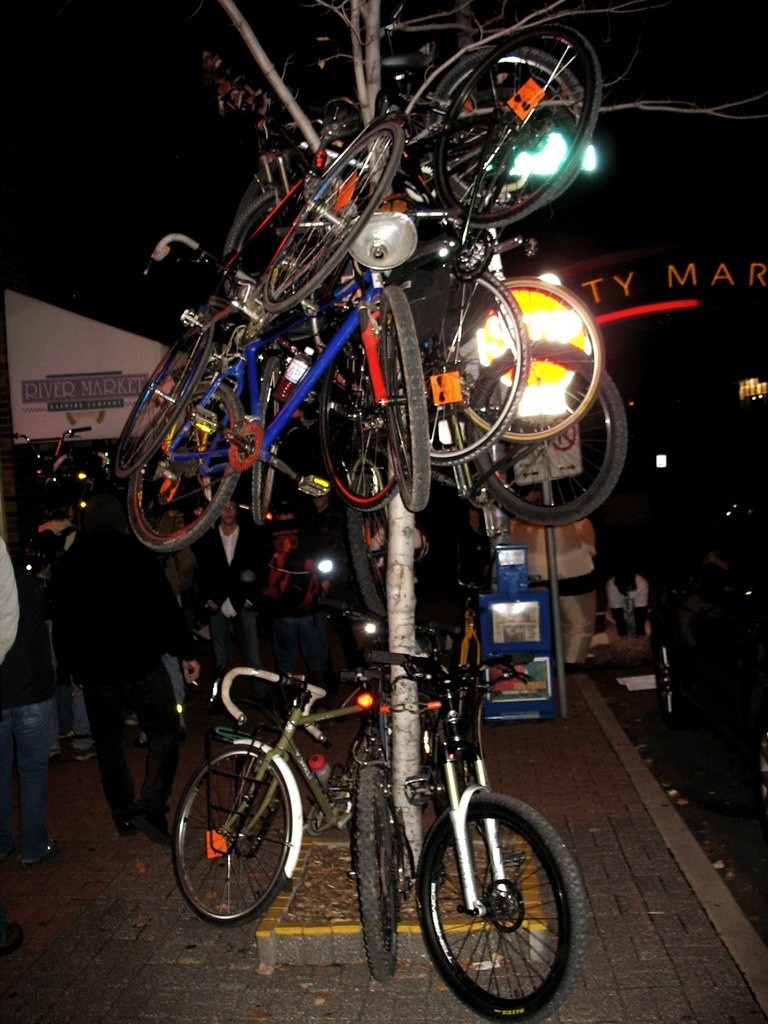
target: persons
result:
[362,516,442,662]
[458,502,491,593]
[25,495,202,843]
[605,566,653,639]
[205,493,269,705]
[0,536,24,959]
[0,533,57,867]
[270,492,369,697]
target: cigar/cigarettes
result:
[191,680,199,686]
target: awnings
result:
[2,287,192,443]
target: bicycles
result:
[113,25,627,553]
[173,600,587,1024]
[1,426,93,519]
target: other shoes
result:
[0,920,23,956]
[47,748,61,761]
[116,818,136,835]
[131,808,173,845]
[0,845,17,865]
[70,740,97,761]
[22,839,57,866]
[250,686,267,703]
